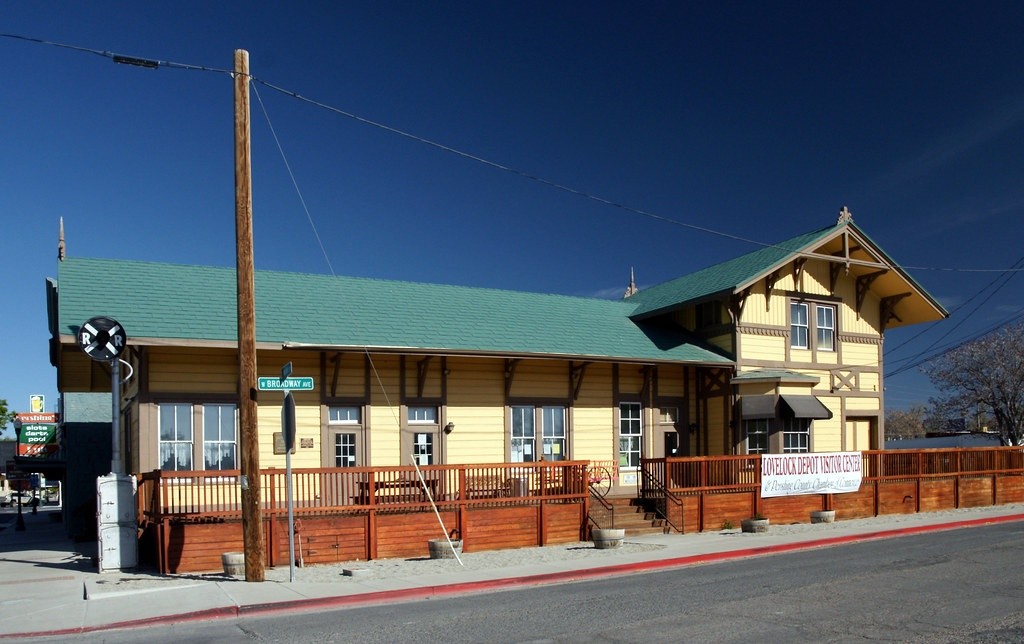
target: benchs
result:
[350,493,450,504]
[458,475,511,500]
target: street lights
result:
[14,417,22,514]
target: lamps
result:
[445,422,455,431]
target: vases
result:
[221,552,245,575]
[427,538,464,558]
[810,510,835,524]
[592,528,625,549]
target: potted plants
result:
[741,512,769,532]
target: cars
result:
[0,492,38,508]
[35,493,40,498]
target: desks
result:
[356,479,439,505]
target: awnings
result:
[780,395,833,418]
[742,395,774,420]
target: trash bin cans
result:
[510,478,529,497]
